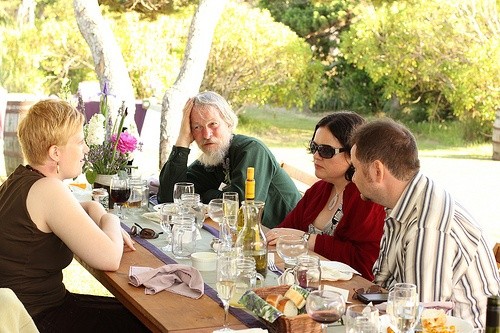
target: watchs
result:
[300,232,311,244]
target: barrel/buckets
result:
[4,100,37,179]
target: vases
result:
[92,172,119,198]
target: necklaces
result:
[327,182,351,209]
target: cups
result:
[345,283,461,333]
[227,257,264,308]
[93,177,239,257]
[190,252,218,272]
[293,255,321,290]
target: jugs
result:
[237,201,269,280]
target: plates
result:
[152,202,211,218]
[143,211,176,224]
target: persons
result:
[263,111,393,281]
[351,119,500,333]
[158,90,303,230]
[0,98,151,333]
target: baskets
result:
[249,285,322,333]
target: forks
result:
[267,260,284,275]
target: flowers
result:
[75,81,142,184]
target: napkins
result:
[375,302,455,315]
[128,264,204,299]
[307,261,362,282]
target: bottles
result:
[483,296,499,333]
[236,167,267,270]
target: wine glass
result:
[275,235,309,271]
[110,178,128,219]
[213,263,238,333]
[208,199,225,241]
[304,290,345,332]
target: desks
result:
[73,200,388,333]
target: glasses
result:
[129,223,163,239]
[310,141,348,159]
